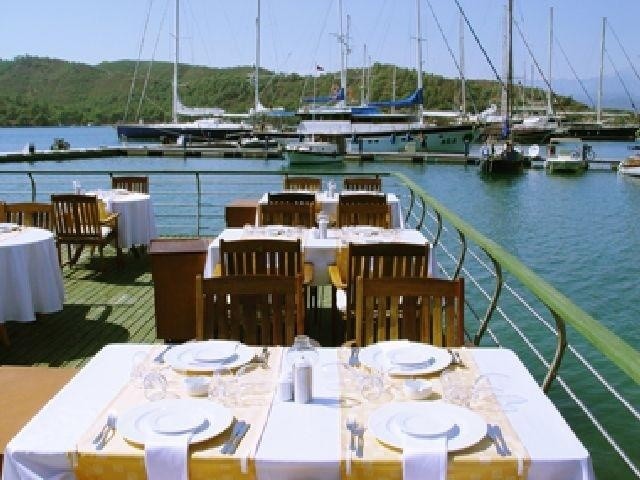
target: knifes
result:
[228,424,250,454]
[221,421,244,452]
[487,424,503,456]
[494,425,512,457]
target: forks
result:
[92,414,113,444]
[96,420,116,451]
[346,416,357,450]
[355,423,365,458]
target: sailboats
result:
[119,0,476,152]
[423,34,639,142]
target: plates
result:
[191,347,237,363]
[155,413,208,433]
[400,411,458,437]
[368,400,487,454]
[390,349,437,366]
[164,340,254,372]
[121,399,233,446]
[358,339,453,377]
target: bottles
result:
[280,379,292,402]
[292,364,313,403]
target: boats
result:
[283,141,346,163]
[618,154,640,177]
[544,136,589,173]
[480,143,524,174]
